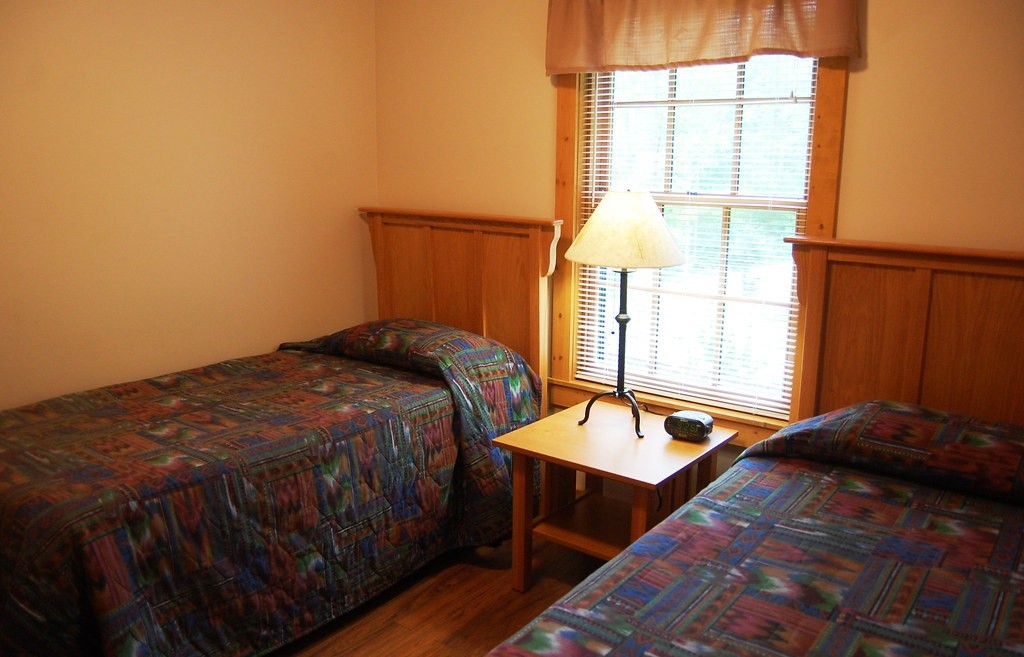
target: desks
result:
[492,397,739,594]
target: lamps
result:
[563,193,687,440]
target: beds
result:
[482,236,1024,657]
[0,208,566,657]
